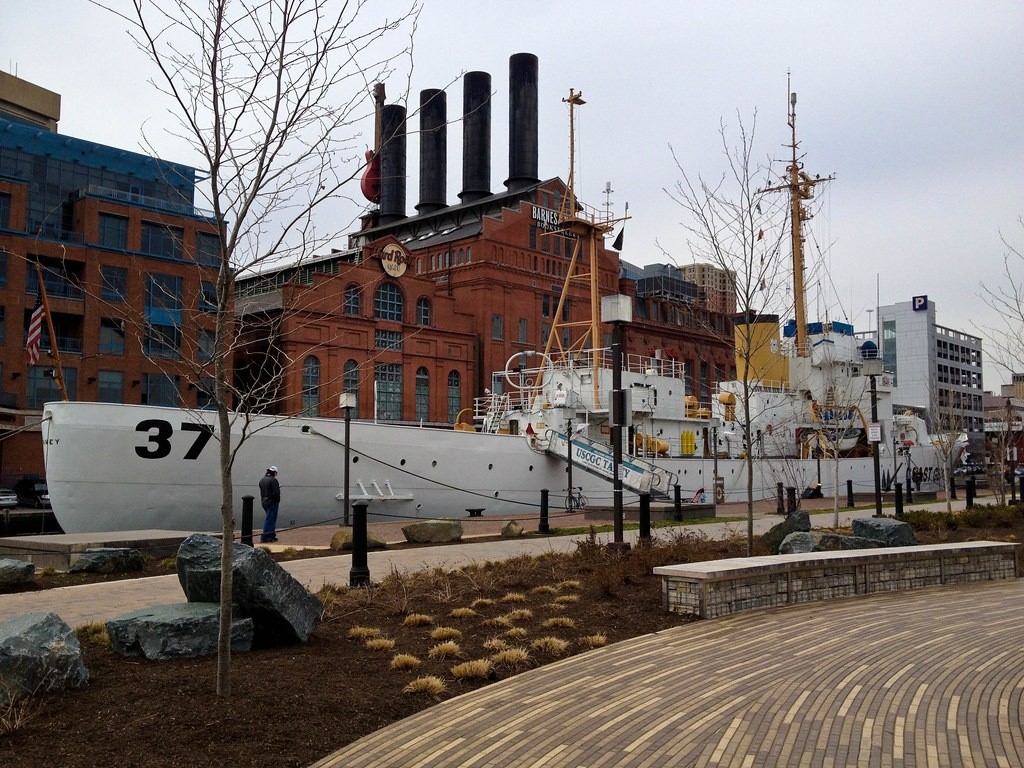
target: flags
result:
[612,227,624,251]
[24,283,47,367]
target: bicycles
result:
[563,487,589,513]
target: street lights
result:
[563,408,576,513]
[813,422,823,498]
[339,392,356,529]
[890,427,898,483]
[710,417,720,505]
[601,294,632,561]
[863,357,887,519]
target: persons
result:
[913,467,923,491]
[259,465,280,543]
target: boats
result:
[42,63,967,535]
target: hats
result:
[270,466,278,473]
